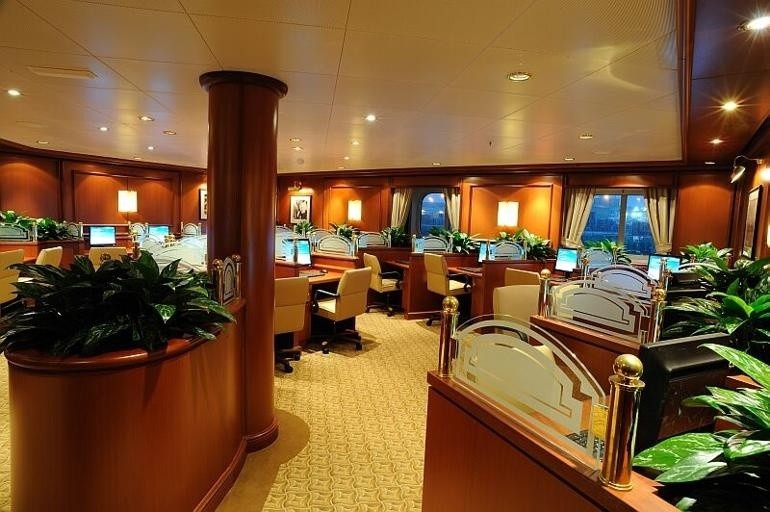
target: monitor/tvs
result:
[89,225,117,246]
[415,238,425,253]
[140,224,171,246]
[657,288,707,341]
[477,240,496,264]
[281,238,312,268]
[647,252,683,285]
[666,271,702,291]
[637,328,732,457]
[554,246,581,280]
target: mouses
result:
[320,269,328,273]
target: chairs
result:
[0,248,24,313]
[89,247,127,272]
[35,246,63,269]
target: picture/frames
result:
[290,195,312,224]
[199,190,208,221]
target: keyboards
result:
[565,428,606,460]
[299,271,324,276]
[456,266,481,273]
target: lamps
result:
[728,155,763,184]
[119,191,139,235]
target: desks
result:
[24,257,36,267]
[529,393,613,462]
